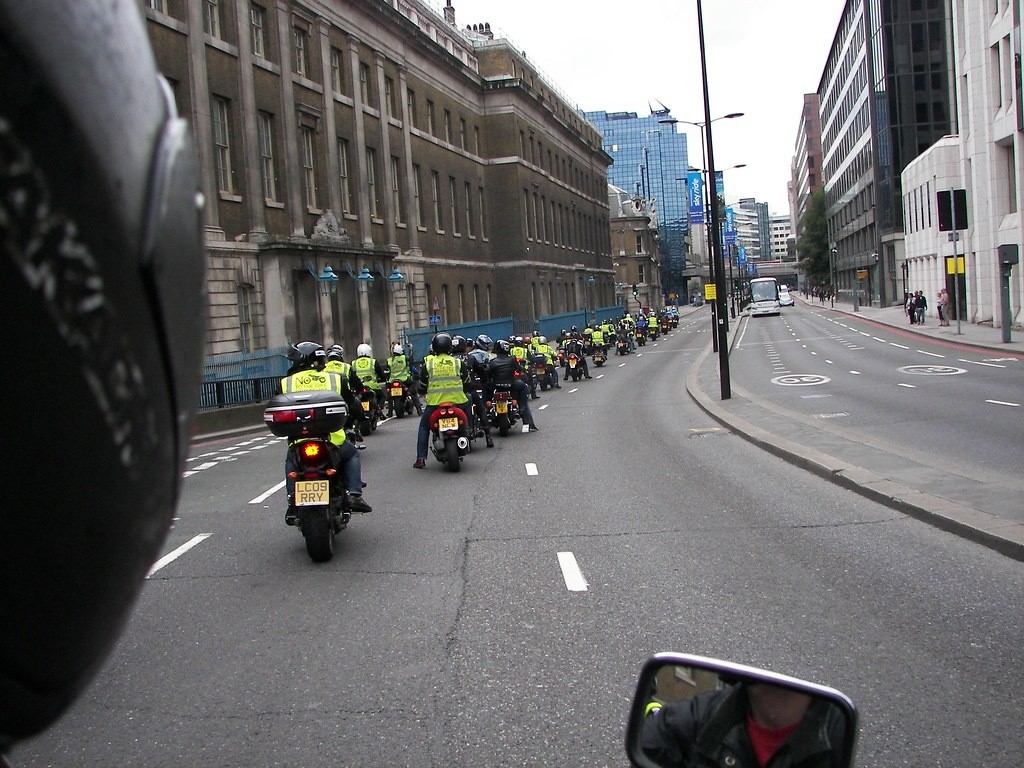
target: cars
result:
[779,293,794,306]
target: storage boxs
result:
[263,391,350,437]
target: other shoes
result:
[532,376,593,399]
[528,425,538,431]
[352,428,363,442]
[378,411,386,420]
[418,411,424,416]
[487,438,495,447]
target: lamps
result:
[300,262,339,282]
[344,265,375,282]
[378,262,404,281]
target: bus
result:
[748,277,781,316]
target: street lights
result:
[720,222,752,319]
[659,112,745,400]
[687,165,747,332]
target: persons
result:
[906,288,951,327]
[275,305,682,526]
[802,285,834,301]
[640,669,851,767]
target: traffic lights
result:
[633,285,637,296]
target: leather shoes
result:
[346,496,372,512]
[413,459,426,468]
[285,505,296,526]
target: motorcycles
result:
[263,386,378,562]
[464,308,680,433]
[343,364,420,440]
[411,371,484,471]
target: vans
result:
[776,285,788,294]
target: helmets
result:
[0,0,210,755]
[509,307,675,344]
[357,344,371,358]
[390,343,402,355]
[476,334,492,351]
[465,337,474,347]
[326,344,344,361]
[429,344,436,354]
[453,336,466,353]
[496,341,510,354]
[294,342,325,362]
[432,332,451,353]
